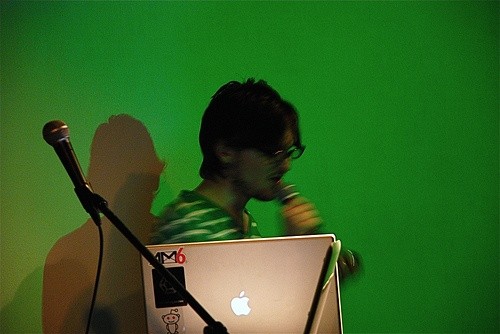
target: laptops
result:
[143,234,344,334]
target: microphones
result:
[279,182,300,205]
[43,120,101,226]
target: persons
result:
[150,78,324,246]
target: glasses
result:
[242,142,306,161]
